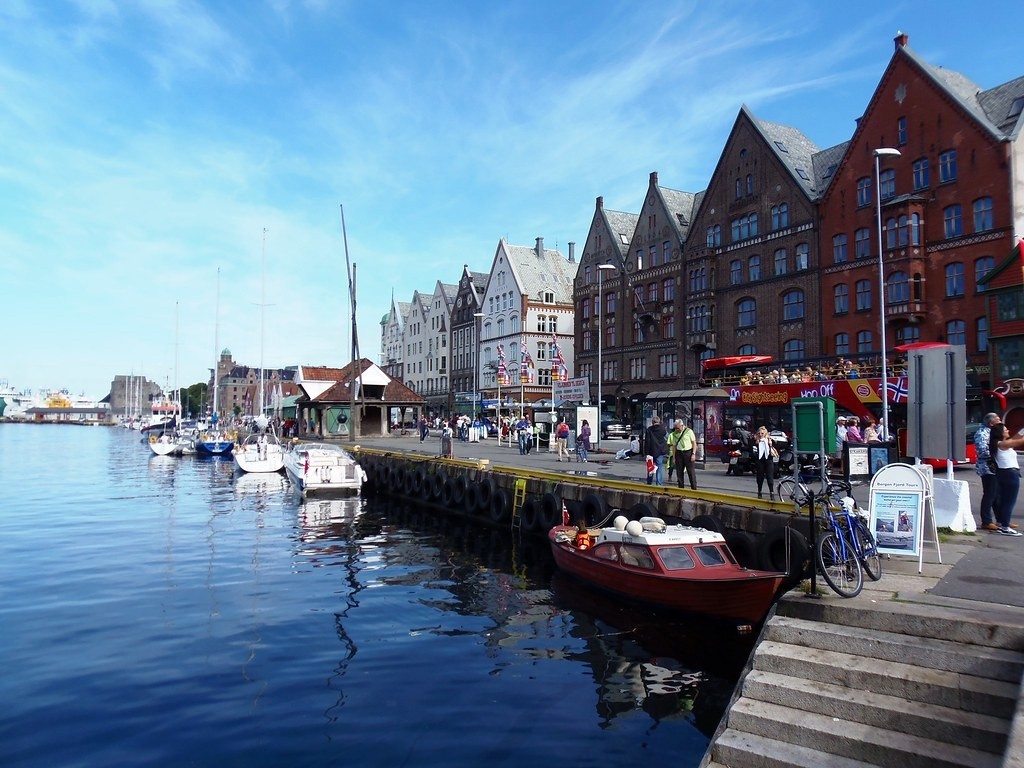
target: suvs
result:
[602,410,632,439]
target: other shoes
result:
[839,470,843,474]
[770,492,776,501]
[556,459,562,462]
[981,522,999,529]
[726,471,731,474]
[997,522,1018,528]
[758,494,762,500]
[624,456,630,459]
[668,478,673,481]
[569,456,571,462]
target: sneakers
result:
[997,526,1003,533]
[1001,527,1023,536]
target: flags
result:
[305,451,309,474]
[497,347,511,385]
[562,503,569,524]
[552,332,568,381]
[520,342,534,383]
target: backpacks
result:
[558,423,567,437]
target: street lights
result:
[597,264,616,449]
[872,148,901,444]
[473,313,485,420]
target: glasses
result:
[758,433,764,434]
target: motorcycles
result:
[723,439,759,476]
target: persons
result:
[712,356,908,386]
[835,415,893,474]
[750,426,780,500]
[974,412,1024,536]
[428,415,446,429]
[500,413,519,439]
[393,416,398,430]
[310,418,315,432]
[576,437,585,462]
[277,417,299,438]
[515,415,533,455]
[645,417,697,490]
[303,419,307,431]
[555,416,571,462]
[624,436,639,460]
[450,414,471,442]
[576,419,591,463]
[418,414,427,443]
[472,413,496,431]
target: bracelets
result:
[693,452,695,455]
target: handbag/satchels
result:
[986,456,996,473]
[662,445,670,454]
[646,455,658,476]
[770,446,778,456]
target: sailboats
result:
[120,371,148,430]
[147,228,292,473]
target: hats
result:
[838,416,847,421]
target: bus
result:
[700,355,1007,470]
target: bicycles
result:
[777,455,891,598]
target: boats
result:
[549,497,788,623]
[282,441,368,498]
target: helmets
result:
[732,419,741,427]
[741,421,746,427]
